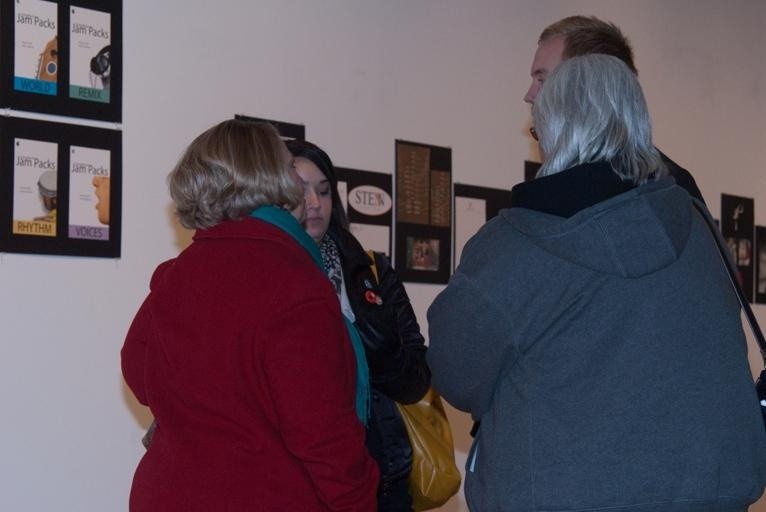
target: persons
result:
[118,119,380,512]
[738,239,752,266]
[520,11,710,210]
[277,138,432,511]
[424,51,766,512]
[91,177,111,227]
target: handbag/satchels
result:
[395,384,461,511]
